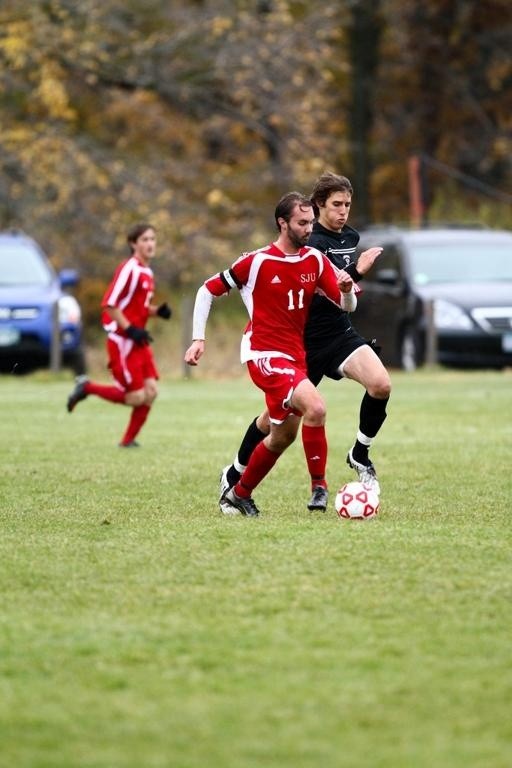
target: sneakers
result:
[218,465,260,517]
[346,445,381,495]
[68,376,90,412]
[308,488,328,511]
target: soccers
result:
[334,483,379,520]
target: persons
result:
[68,224,171,448]
[186,191,361,516]
[219,171,389,491]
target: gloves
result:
[156,302,171,320]
[124,323,154,347]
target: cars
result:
[351,222,512,372]
[0,226,89,376]
